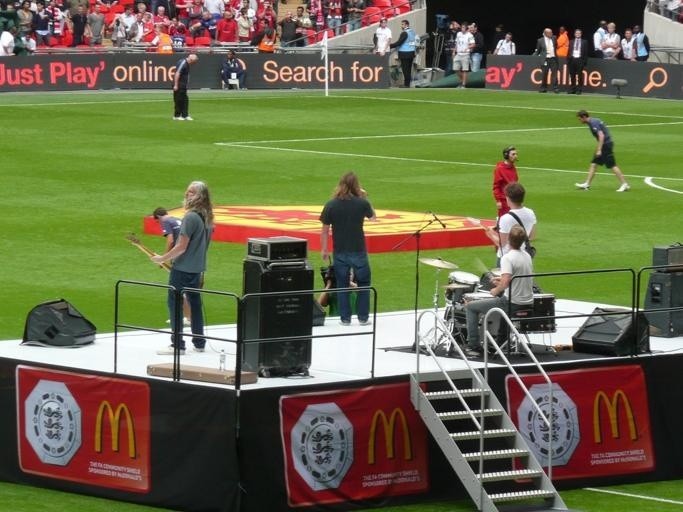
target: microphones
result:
[427,206,448,231]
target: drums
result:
[520,294,555,332]
[445,284,470,304]
[462,292,494,305]
[491,267,502,278]
[448,271,481,292]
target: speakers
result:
[240,258,316,377]
[21,296,101,349]
[572,306,650,357]
[642,272,682,342]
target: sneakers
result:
[337,319,352,326]
[451,345,482,357]
[182,116,193,120]
[156,345,185,355]
[190,338,205,352]
[575,182,591,190]
[359,320,372,325]
[616,183,630,192]
[173,116,185,120]
[461,85,465,88]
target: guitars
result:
[125,236,203,288]
[467,215,535,259]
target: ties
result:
[576,38,578,50]
[605,30,607,34]
[635,35,637,38]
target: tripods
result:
[384,231,433,357]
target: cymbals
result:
[418,258,458,269]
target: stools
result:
[222,79,239,90]
[509,312,532,351]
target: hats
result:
[546,38,550,55]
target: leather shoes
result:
[552,88,560,93]
[539,88,547,92]
[576,90,581,95]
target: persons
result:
[633,24,650,62]
[0,0,365,54]
[390,20,417,88]
[317,264,359,317]
[153,207,204,328]
[493,32,516,55]
[574,109,631,192]
[555,27,570,93]
[319,170,377,326]
[173,53,200,121]
[600,22,622,61]
[491,24,507,54]
[567,28,588,95]
[442,20,462,76]
[467,23,485,72]
[463,223,533,356]
[451,21,476,88]
[372,16,392,56]
[221,50,248,91]
[592,20,609,58]
[620,28,637,61]
[492,145,519,219]
[484,182,538,271]
[151,181,216,353]
[532,28,561,93]
